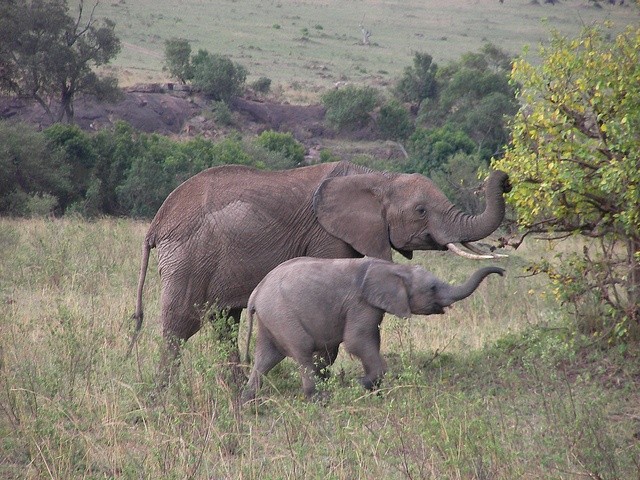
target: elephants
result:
[242,257,507,404]
[123,159,513,389]
